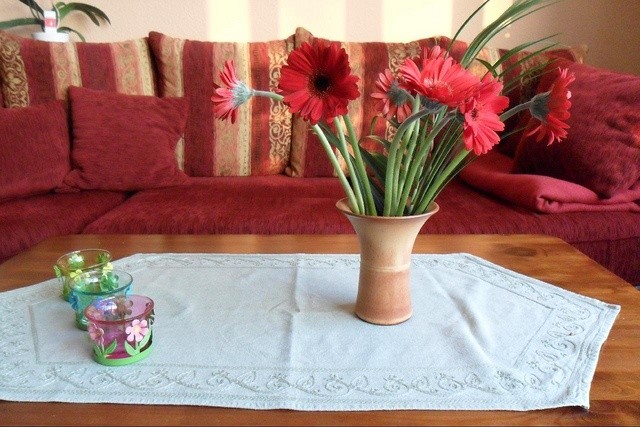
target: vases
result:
[335,195,439,327]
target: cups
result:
[54,248,113,304]
[68,268,134,332]
[85,295,156,366]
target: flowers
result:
[212,0,575,215]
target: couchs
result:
[1,29,640,281]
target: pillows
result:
[0,101,74,198]
[512,54,640,199]
[65,85,193,192]
